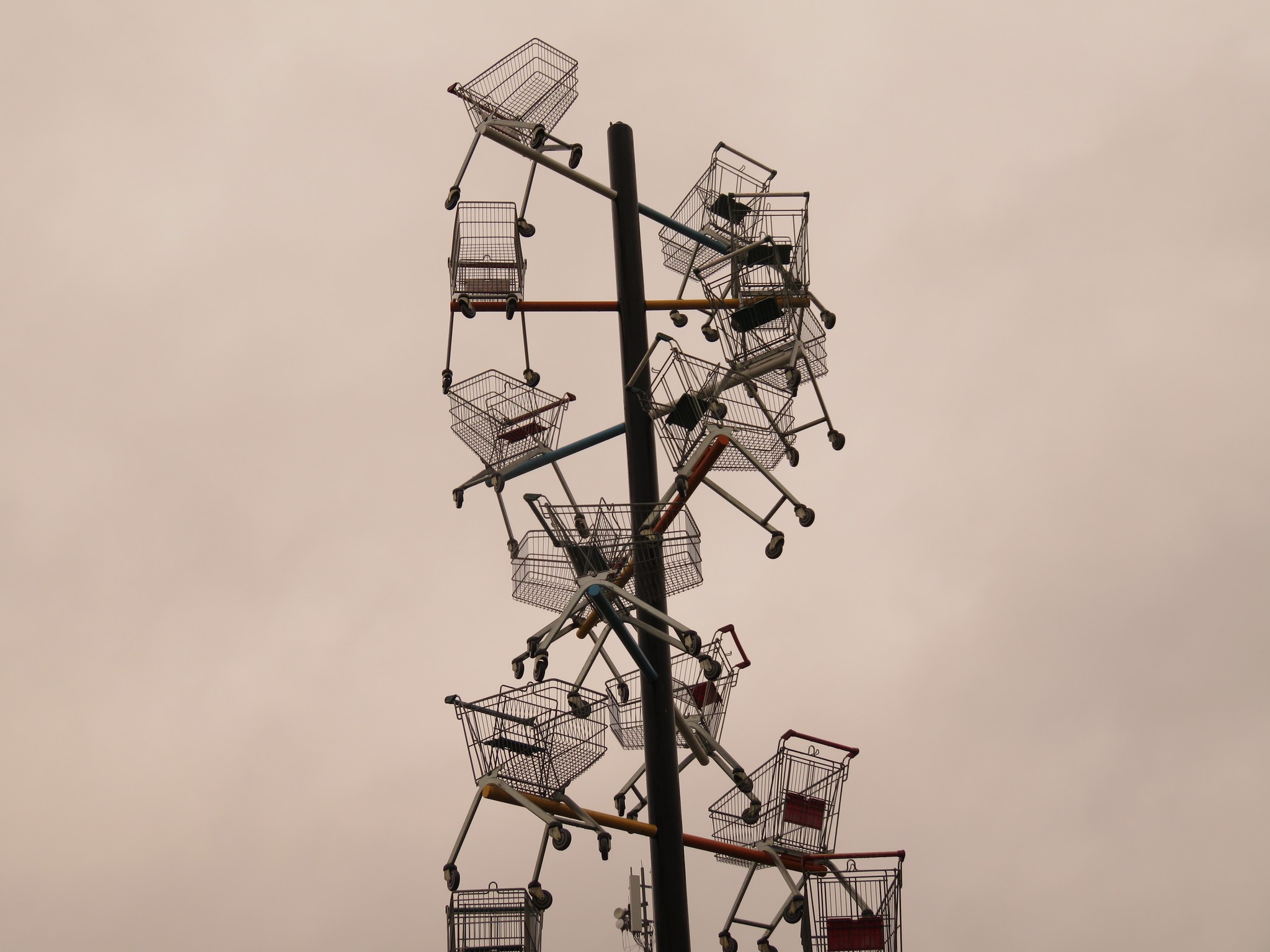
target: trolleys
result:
[686,235,842,467]
[656,141,777,346]
[441,200,542,397]
[510,529,700,679]
[442,881,547,951]
[703,190,837,341]
[444,36,581,237]
[624,329,815,559]
[705,724,874,950]
[600,624,759,826]
[787,849,906,952]
[440,676,612,889]
[525,485,719,718]
[444,369,591,557]
